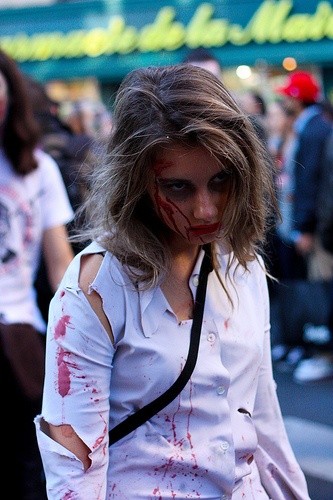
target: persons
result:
[24,79,89,329]
[269,69,333,385]
[182,49,223,87]
[237,84,265,122]
[31,63,316,500]
[251,98,308,370]
[0,50,80,500]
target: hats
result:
[275,70,322,103]
